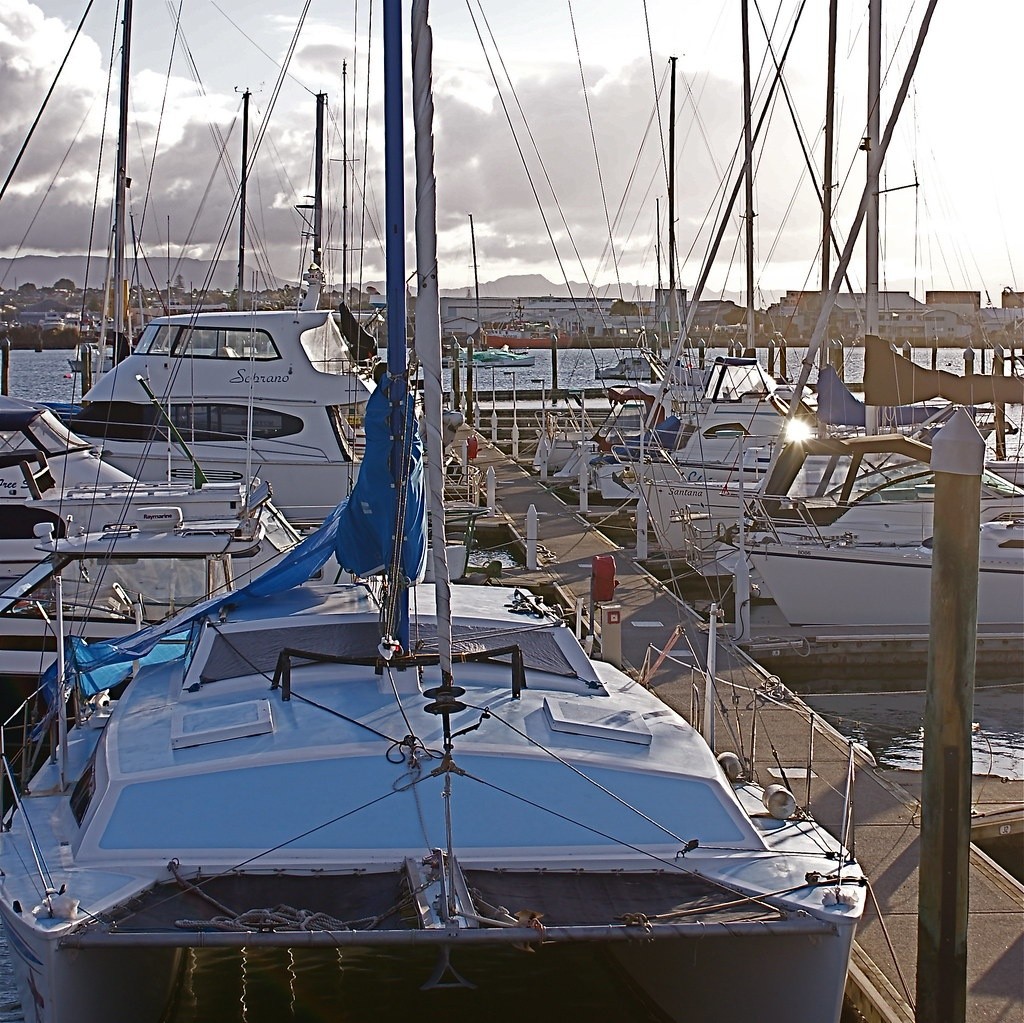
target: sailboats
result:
[0,2,1023,1023]
